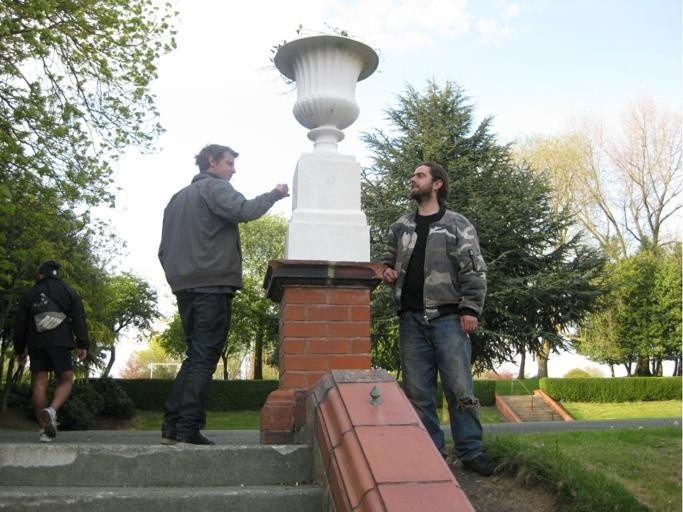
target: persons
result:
[379,161,495,477]
[158,145,289,445]
[13,260,90,442]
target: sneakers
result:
[40,405,58,438]
[159,429,177,445]
[464,452,498,478]
[176,430,215,446]
[38,429,53,442]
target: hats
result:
[38,260,64,281]
